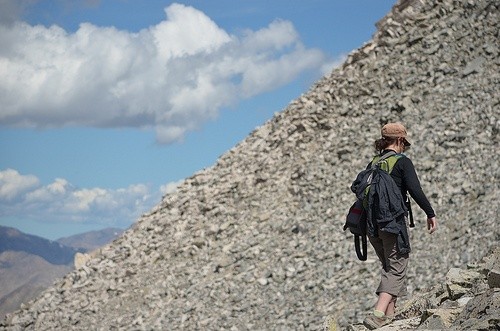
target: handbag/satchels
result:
[342,200,366,236]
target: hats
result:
[382,124,412,146]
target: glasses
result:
[401,142,406,152]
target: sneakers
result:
[364,313,385,330]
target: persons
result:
[344,122,436,329]
